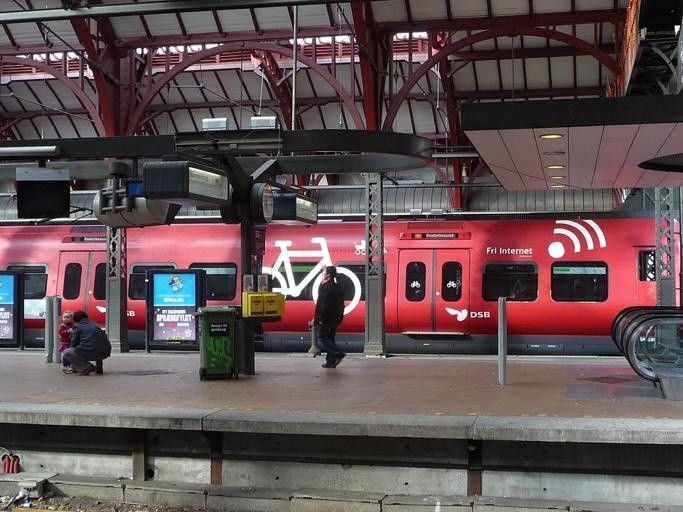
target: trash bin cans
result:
[194,306,239,381]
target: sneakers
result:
[81,367,95,375]
[96,360,103,373]
[334,352,345,365]
[322,364,335,368]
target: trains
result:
[0,183,682,358]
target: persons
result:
[57,310,75,374]
[313,266,345,368]
[62,309,112,375]
